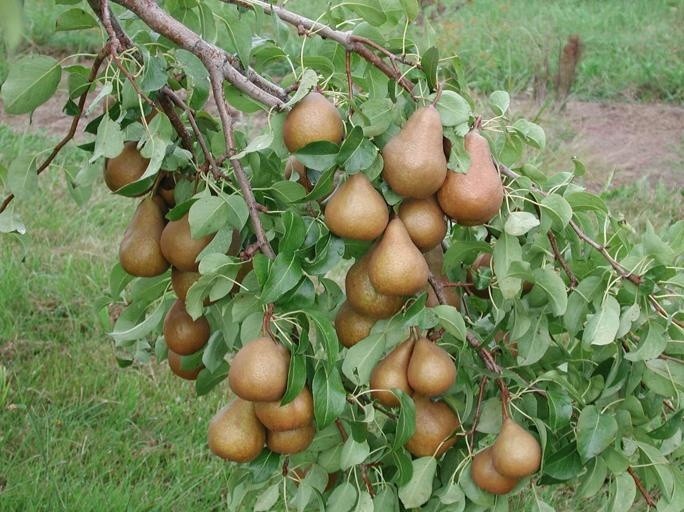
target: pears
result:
[106,89,541,495]
